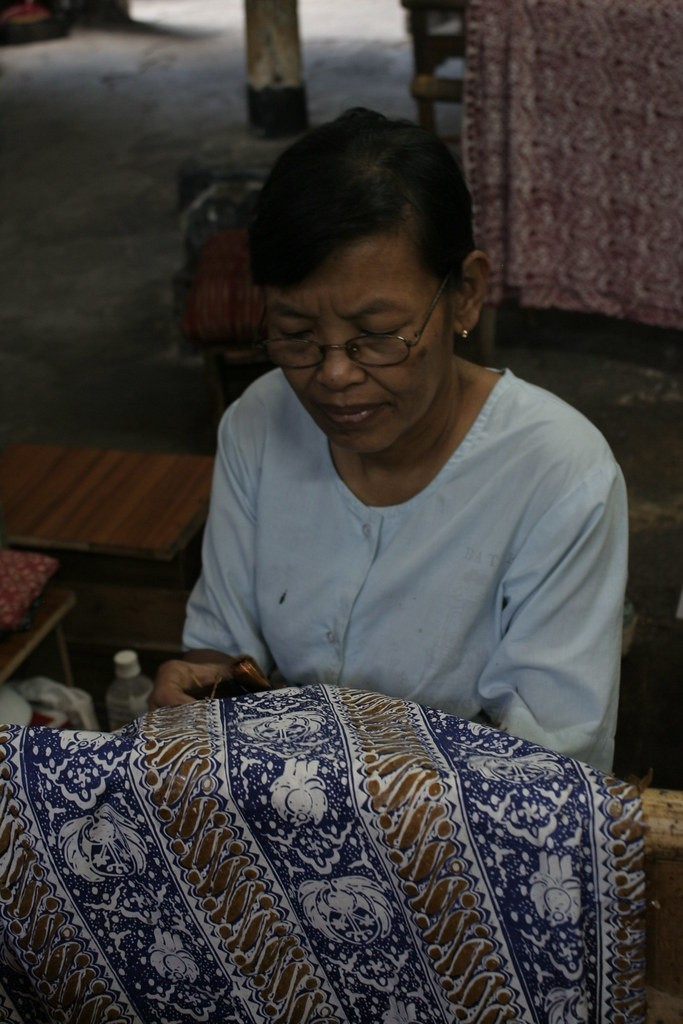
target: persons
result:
[150,106,628,773]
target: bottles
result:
[106,650,154,732]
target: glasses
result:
[252,266,456,369]
